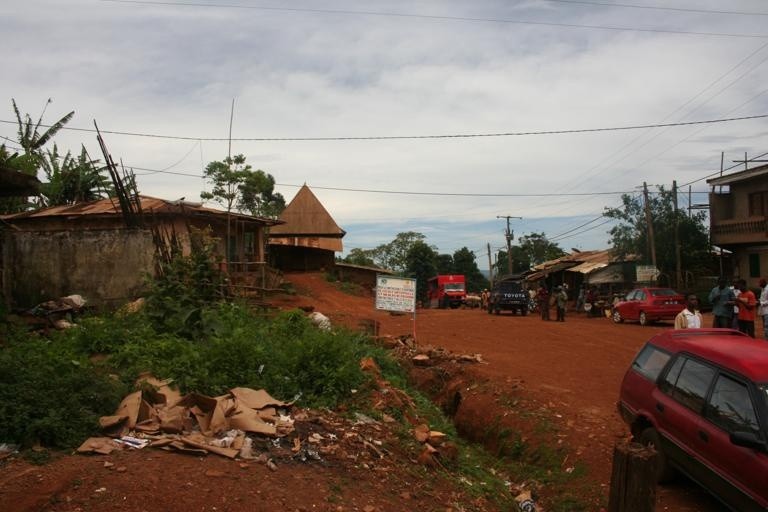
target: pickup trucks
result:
[487,281,530,315]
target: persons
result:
[733,279,758,339]
[480,282,586,322]
[707,274,737,328]
[672,291,703,330]
[755,278,768,340]
[727,275,741,330]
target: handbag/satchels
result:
[733,306,739,314]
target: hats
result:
[558,285,564,290]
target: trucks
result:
[428,272,467,314]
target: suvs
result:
[617,328,767,510]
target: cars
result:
[613,287,688,326]
